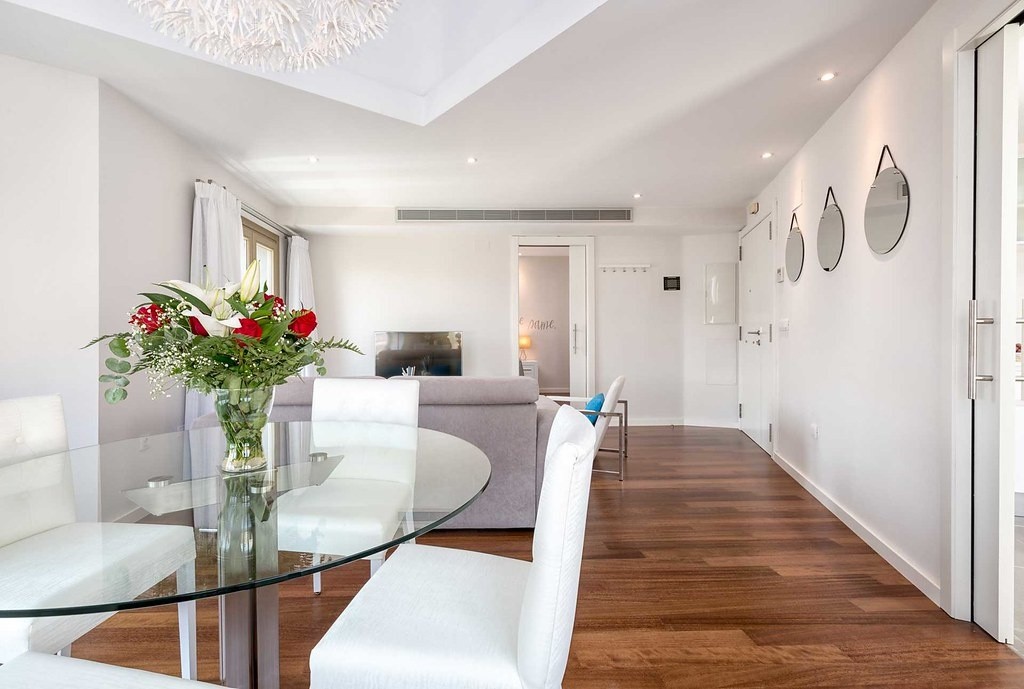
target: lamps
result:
[518,335,532,360]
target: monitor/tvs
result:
[374,330,463,379]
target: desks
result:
[0,419,492,689]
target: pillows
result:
[583,392,605,424]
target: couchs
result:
[186,375,562,533]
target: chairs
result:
[310,402,596,688]
[0,396,196,681]
[548,375,628,482]
[276,378,423,595]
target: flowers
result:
[80,261,365,406]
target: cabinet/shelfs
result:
[520,360,539,380]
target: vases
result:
[214,384,276,473]
